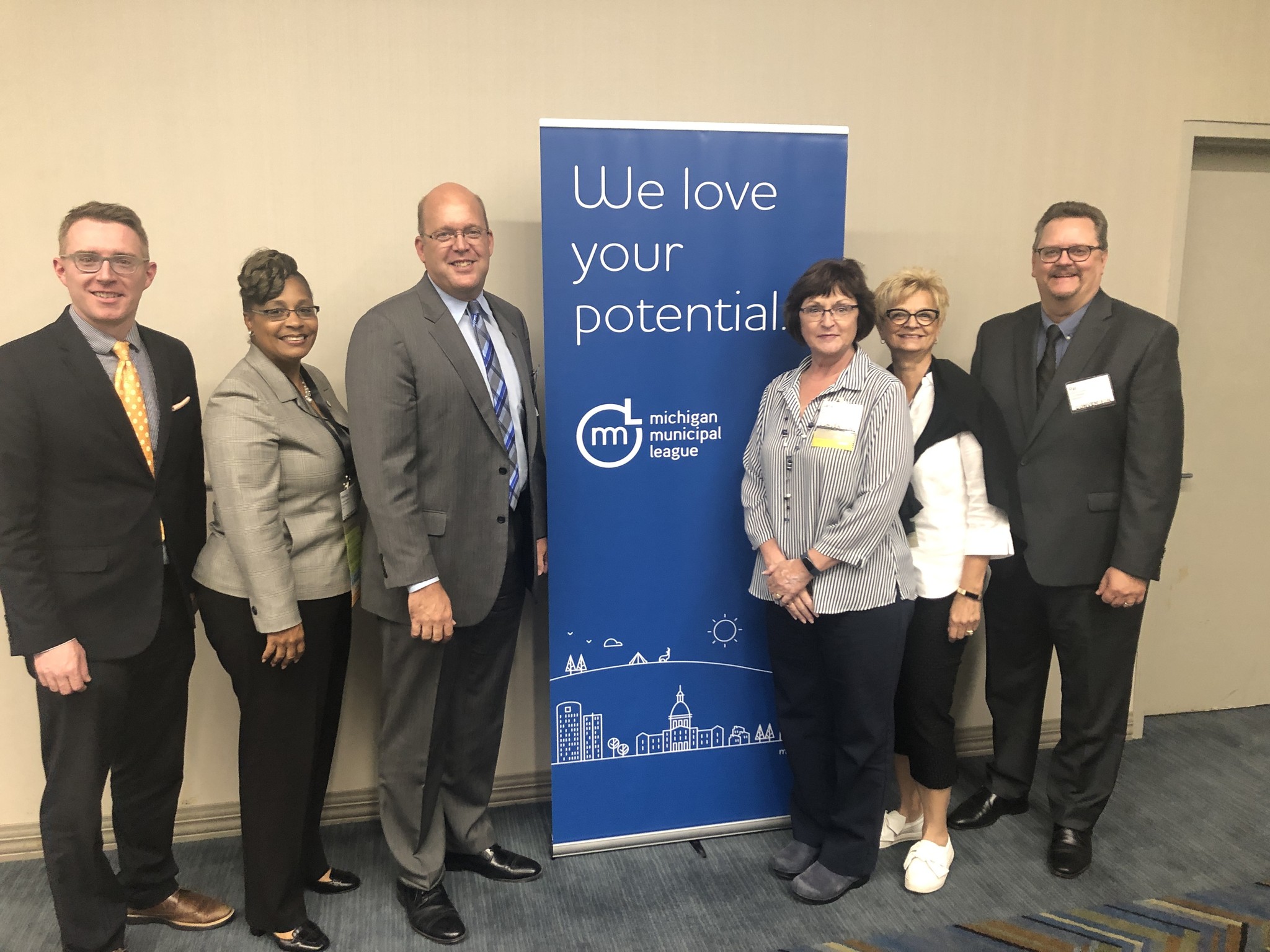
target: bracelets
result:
[956,587,983,601]
[800,551,822,577]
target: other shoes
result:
[768,838,821,879]
[789,861,868,905]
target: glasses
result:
[419,228,489,247]
[1034,244,1101,263]
[59,251,150,275]
[885,307,939,326]
[799,304,859,323]
[245,305,319,321]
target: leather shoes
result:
[126,886,236,931]
[298,864,361,895]
[947,786,1030,831]
[264,919,330,952]
[395,877,466,943]
[1048,822,1093,877]
[444,842,543,883]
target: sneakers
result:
[879,809,925,849]
[903,834,954,893]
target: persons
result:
[192,247,367,952]
[0,202,235,952]
[872,272,1017,895]
[739,259,914,904]
[945,198,1184,879]
[343,182,549,947]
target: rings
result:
[775,593,781,600]
[1124,602,1134,607]
[965,630,974,636]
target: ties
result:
[109,340,166,542]
[465,300,520,512]
[1035,325,1064,412]
